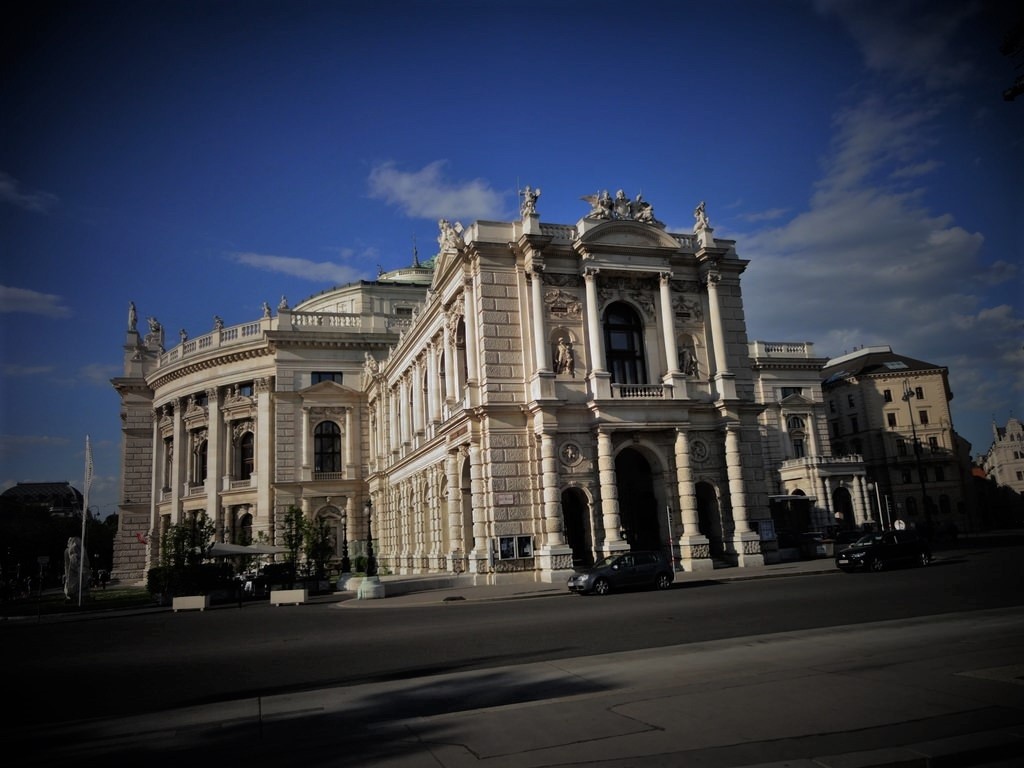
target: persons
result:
[327,561,339,593]
[94,571,108,589]
[214,315,222,329]
[189,395,198,412]
[438,216,454,243]
[279,296,287,308]
[232,384,242,402]
[161,405,169,422]
[555,337,567,373]
[148,317,159,331]
[517,184,535,207]
[127,300,137,330]
[179,329,187,342]
[10,576,32,600]
[696,201,710,228]
[563,446,576,462]
[598,187,655,224]
[262,302,272,318]
[679,341,697,374]
[244,554,259,592]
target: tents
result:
[204,541,296,579]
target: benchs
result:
[173,595,210,612]
[270,589,308,607]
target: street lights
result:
[340,510,351,572]
[901,376,933,527]
[363,499,378,577]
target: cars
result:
[835,528,932,573]
[567,548,677,597]
